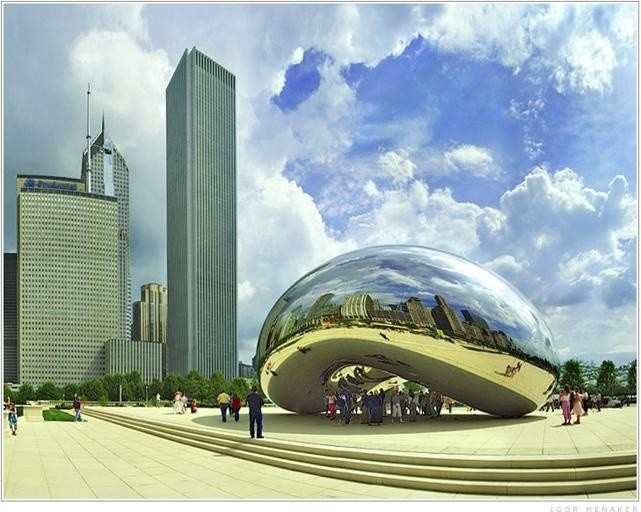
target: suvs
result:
[602,395,622,408]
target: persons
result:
[171,391,188,415]
[244,384,265,439]
[155,393,160,407]
[229,392,234,416]
[570,387,605,412]
[504,362,524,378]
[72,396,83,422]
[446,397,455,413]
[321,385,444,428]
[3,401,17,436]
[544,395,555,412]
[191,399,198,413]
[216,390,231,423]
[559,384,573,426]
[230,394,242,421]
[570,386,585,424]
